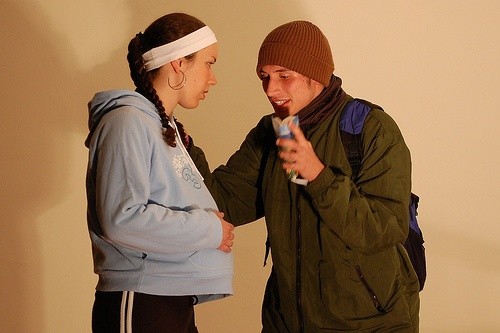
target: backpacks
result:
[339,99,426,295]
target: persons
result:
[84,13,236,333]
[175,20,422,333]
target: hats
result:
[258,21,335,88]
